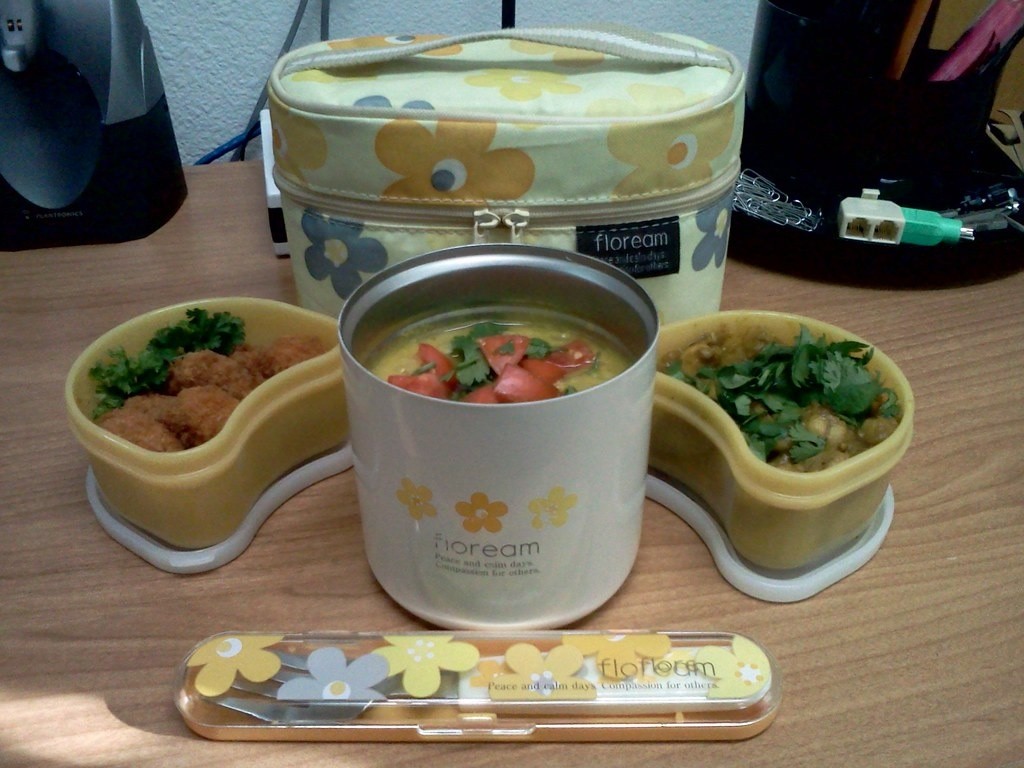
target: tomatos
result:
[367,306,638,405]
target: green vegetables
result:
[662,321,900,467]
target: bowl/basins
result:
[645,311,913,574]
[337,243,661,634]
[62,296,352,550]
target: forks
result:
[203,651,764,727]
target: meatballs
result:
[98,332,329,453]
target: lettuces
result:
[86,306,246,421]
